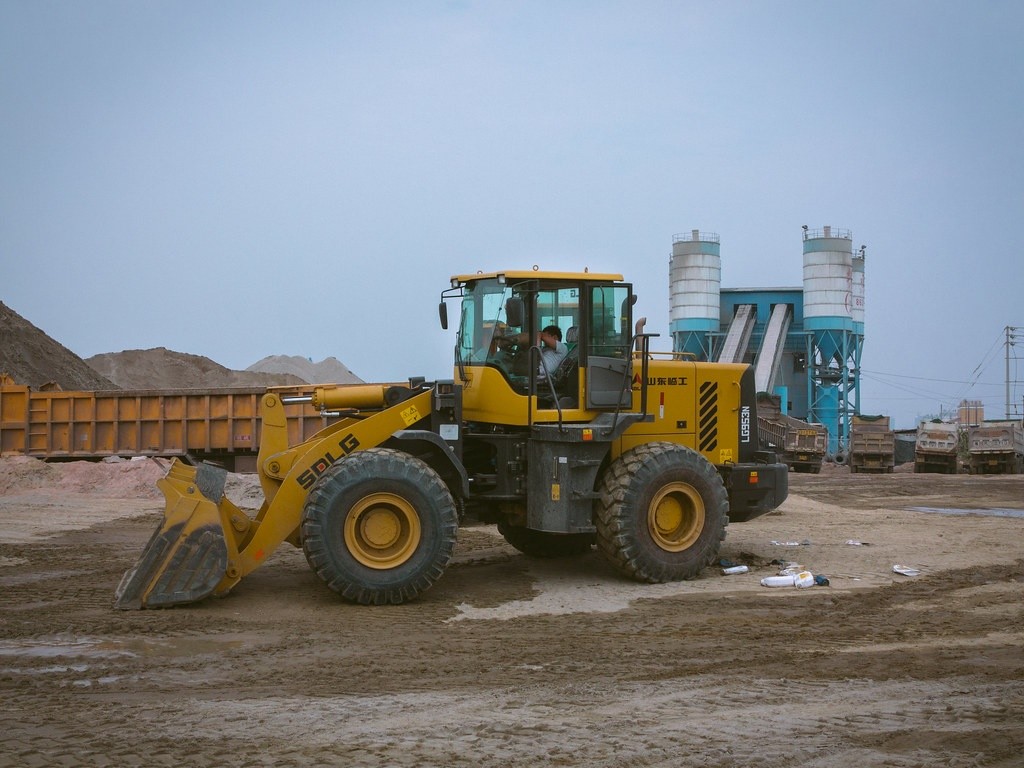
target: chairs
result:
[510,326,580,393]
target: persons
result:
[491,323,568,376]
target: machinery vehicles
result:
[108,262,791,615]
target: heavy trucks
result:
[849,413,896,474]
[913,422,960,475]
[966,423,1024,476]
[756,390,828,473]
